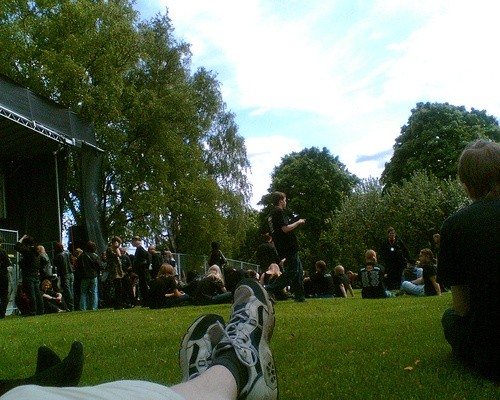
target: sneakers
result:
[177,314,226,381]
[208,276,278,400]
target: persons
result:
[0,341,84,397]
[0,224,452,319]
[264,191,306,303]
[0,277,278,400]
[436,139,500,384]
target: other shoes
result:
[395,292,404,296]
[35,346,61,375]
[58,309,67,313]
[45,341,84,388]
